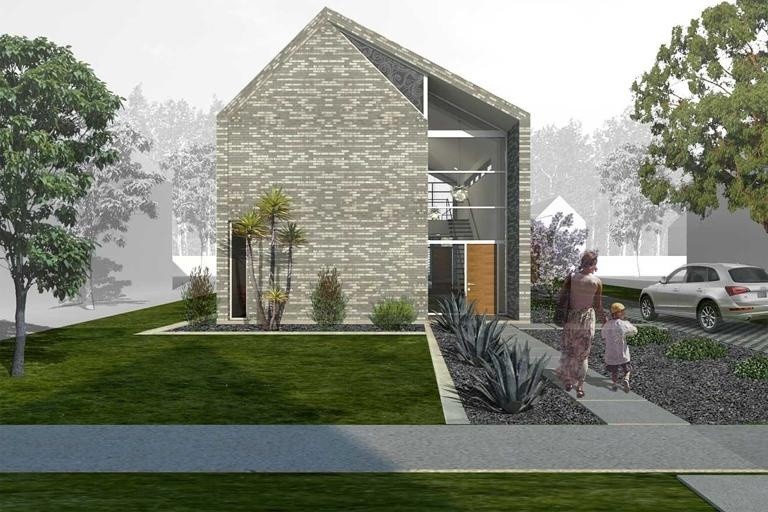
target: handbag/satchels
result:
[552,273,571,327]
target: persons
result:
[557,250,608,398]
[601,301,640,393]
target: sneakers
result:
[623,378,630,393]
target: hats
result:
[610,302,625,315]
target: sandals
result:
[565,384,573,391]
[576,390,584,398]
